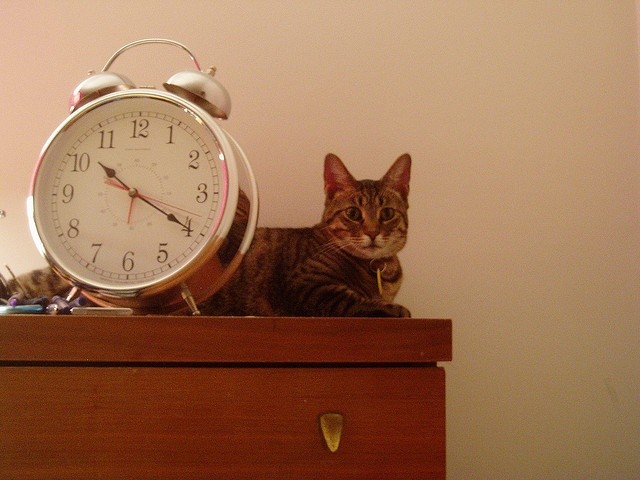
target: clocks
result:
[25,36,259,317]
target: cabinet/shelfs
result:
[1,313,451,479]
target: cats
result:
[1,153,412,318]
